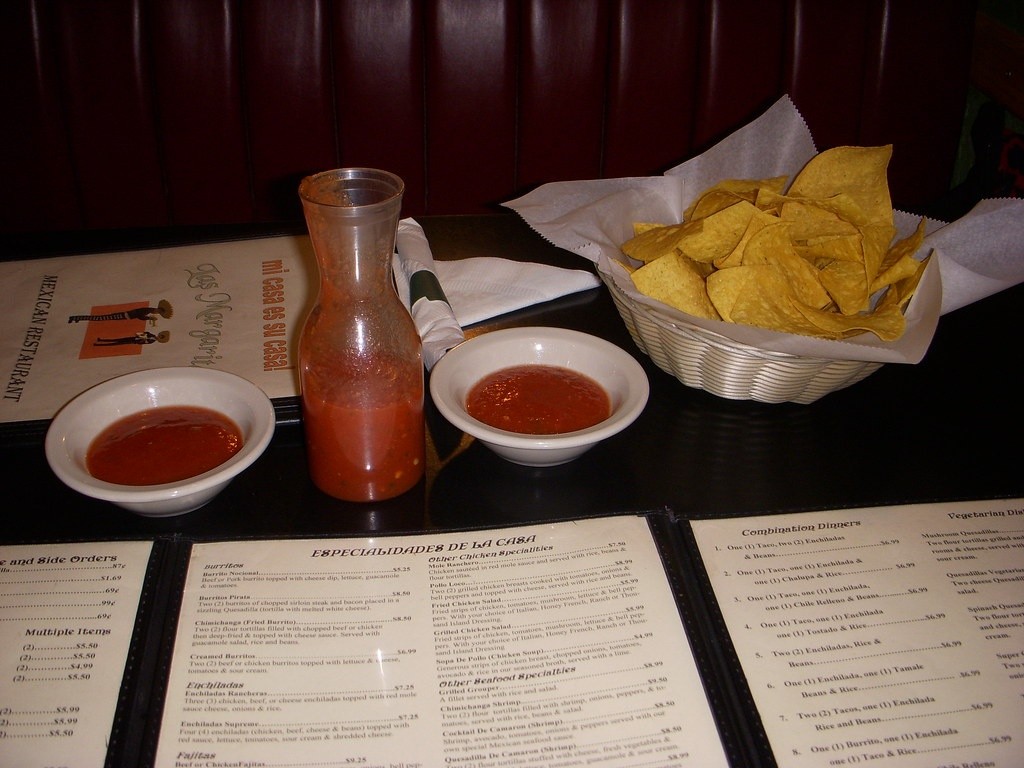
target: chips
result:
[610,144,930,343]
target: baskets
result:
[594,253,889,406]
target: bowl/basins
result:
[45,366,276,517]
[430,327,649,467]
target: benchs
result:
[0,1,976,228]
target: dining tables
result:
[0,214,1024,768]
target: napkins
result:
[391,216,603,375]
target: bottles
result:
[297,168,426,502]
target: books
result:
[0,235,319,425]
